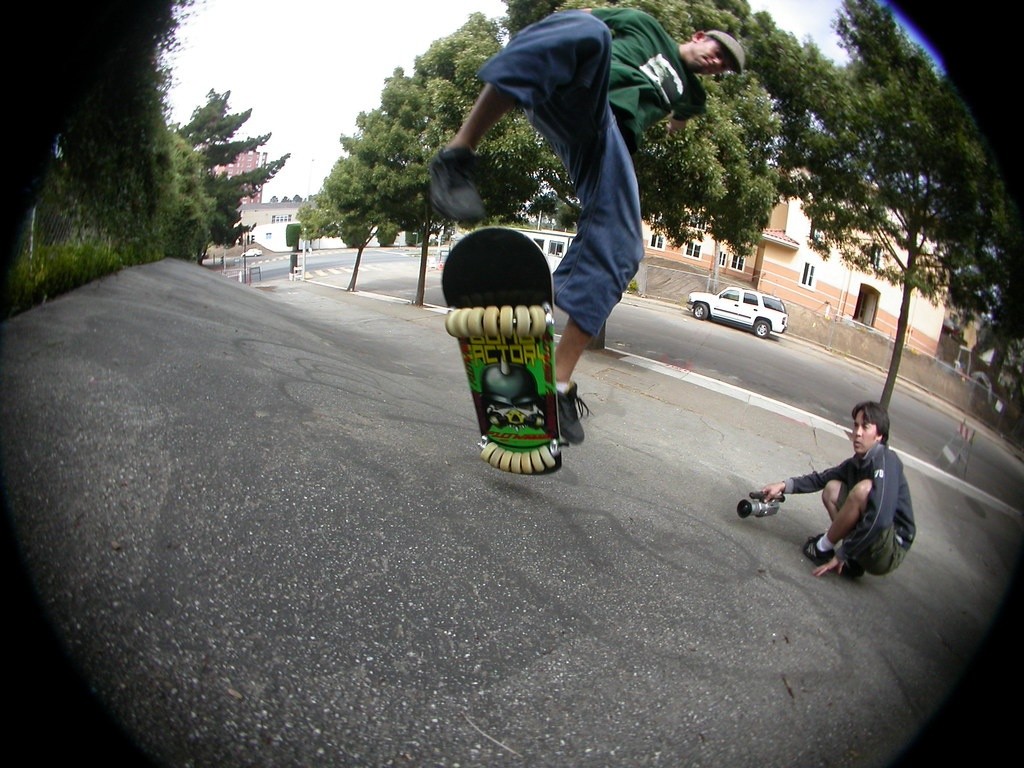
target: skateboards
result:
[441,225,563,478]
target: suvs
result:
[686,286,789,337]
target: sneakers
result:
[842,559,865,578]
[429,146,485,223]
[557,381,585,444]
[803,534,835,567]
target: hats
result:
[705,30,745,74]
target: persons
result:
[424,4,746,446]
[759,400,917,581]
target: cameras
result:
[736,494,786,518]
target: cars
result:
[240,249,263,258]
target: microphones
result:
[750,491,766,500]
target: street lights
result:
[412,233,418,248]
